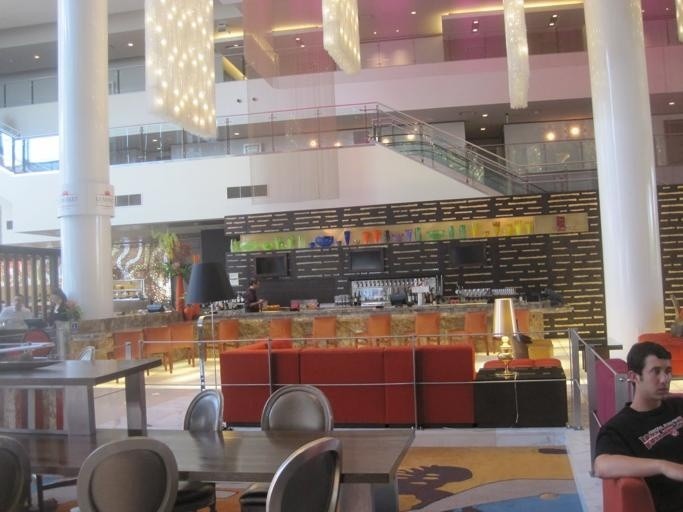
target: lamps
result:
[144,0,216,141]
[492,298,519,380]
[675,0,683,42]
[502,0,529,109]
[322,0,360,75]
[186,263,237,389]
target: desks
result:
[476,368,567,429]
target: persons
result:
[0,294,32,329]
[48,287,79,326]
[591,341,683,512]
[243,277,264,312]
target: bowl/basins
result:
[315,236,334,246]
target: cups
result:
[362,219,534,245]
[230,235,306,253]
[335,231,351,246]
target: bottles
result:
[353,291,362,306]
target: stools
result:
[484,359,561,368]
[638,333,683,377]
